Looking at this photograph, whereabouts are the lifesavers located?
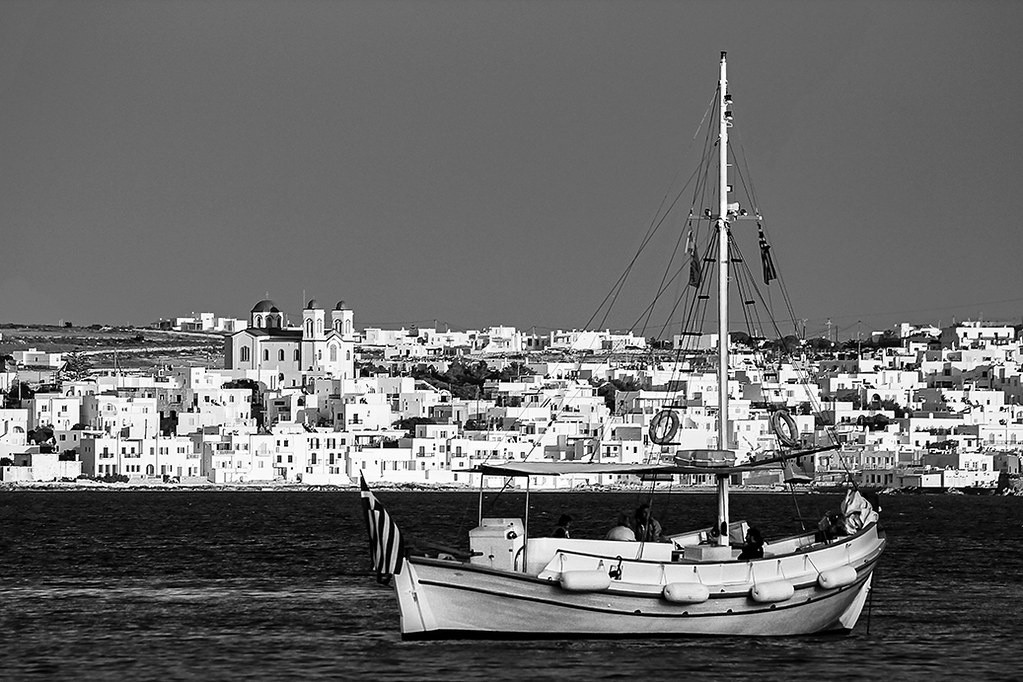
[649,410,679,443]
[773,410,799,445]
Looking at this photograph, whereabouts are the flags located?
[359,477,404,575]
[689,233,703,288]
[758,225,777,285]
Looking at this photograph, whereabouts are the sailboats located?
[358,51,889,641]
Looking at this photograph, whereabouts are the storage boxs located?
[674,450,735,468]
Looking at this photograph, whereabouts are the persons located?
[606,515,636,541]
[737,528,764,561]
[637,504,662,543]
[552,513,574,539]
